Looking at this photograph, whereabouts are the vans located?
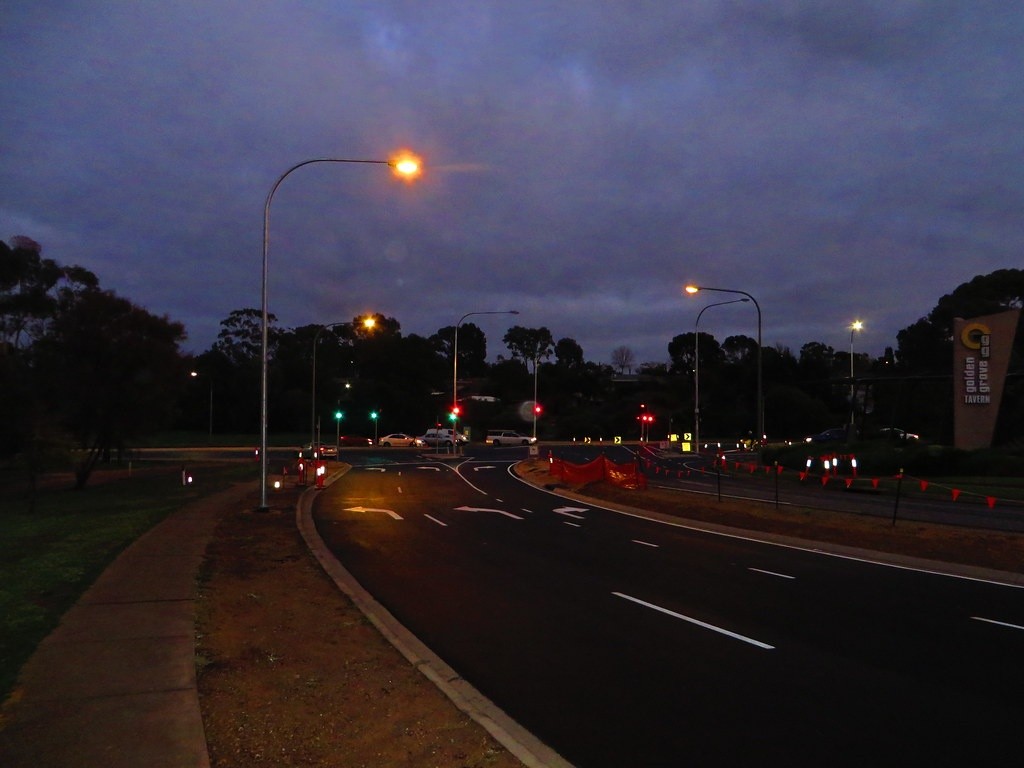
[427,428,469,445]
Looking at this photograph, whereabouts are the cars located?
[299,442,337,458]
[804,428,849,443]
[338,433,375,448]
[379,433,426,448]
[880,428,919,440]
[418,432,457,447]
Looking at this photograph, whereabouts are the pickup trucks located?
[486,431,536,447]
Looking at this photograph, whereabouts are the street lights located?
[310,319,377,461]
[695,298,751,452]
[643,416,653,443]
[335,412,342,443]
[685,285,762,448]
[453,311,519,455]
[191,372,212,436]
[851,322,861,424]
[257,156,417,512]
